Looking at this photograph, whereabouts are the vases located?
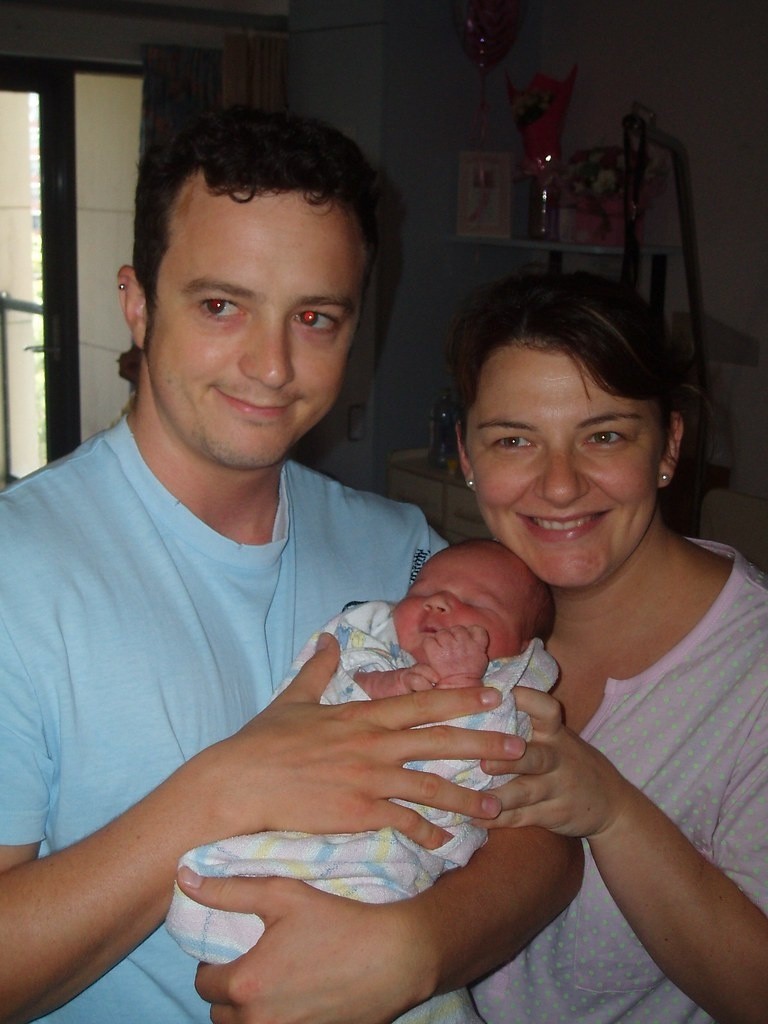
[526,178,558,235]
[575,211,645,246]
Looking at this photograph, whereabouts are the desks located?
[388,448,730,585]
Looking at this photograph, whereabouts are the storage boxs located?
[457,146,529,238]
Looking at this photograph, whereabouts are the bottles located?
[427,388,458,470]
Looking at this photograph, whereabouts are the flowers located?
[560,148,665,215]
[503,64,577,184]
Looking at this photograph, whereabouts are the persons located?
[168,538,564,967]
[0,104,585,1024]
[439,268,768,1024]
